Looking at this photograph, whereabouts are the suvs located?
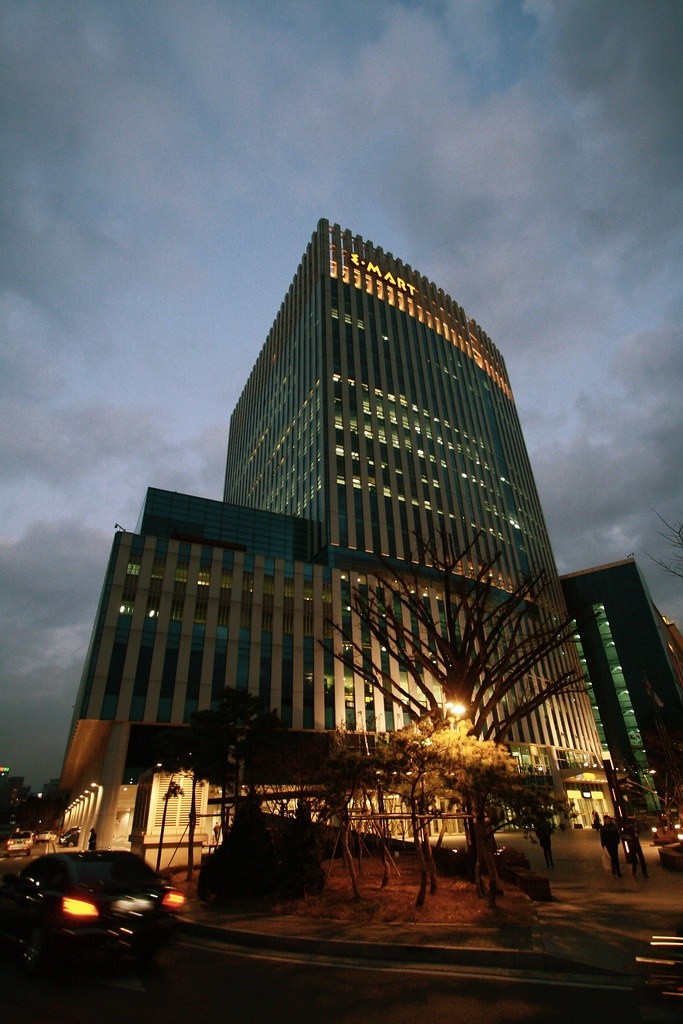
[6,830,33,858]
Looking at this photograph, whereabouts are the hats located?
[623,818,633,824]
[90,828,95,831]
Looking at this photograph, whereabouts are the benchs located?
[515,867,552,901]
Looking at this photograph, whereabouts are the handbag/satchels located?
[626,853,635,863]
[592,823,602,829]
[602,848,611,869]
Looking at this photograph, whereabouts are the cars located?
[59,826,82,848]
[35,831,57,844]
[653,821,683,845]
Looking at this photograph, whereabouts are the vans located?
[0,850,183,966]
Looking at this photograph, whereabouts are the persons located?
[213,822,221,842]
[533,810,652,879]
[88,828,96,850]
